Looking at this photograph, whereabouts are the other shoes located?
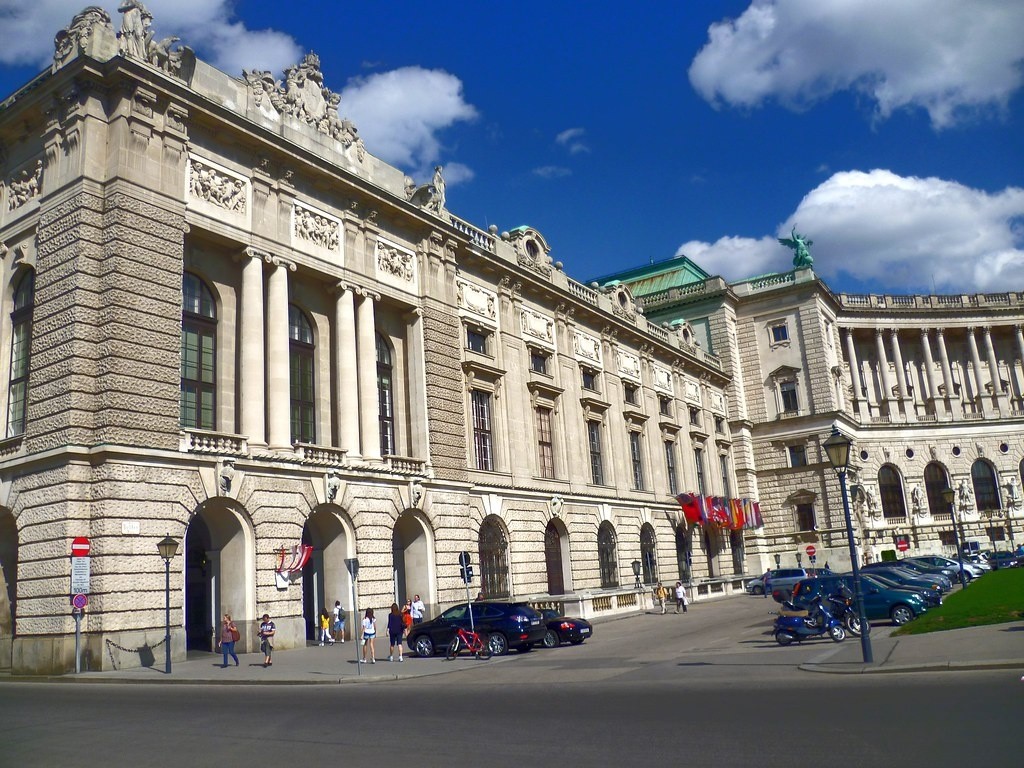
[674,611,679,614]
[684,612,686,613]
[221,665,227,668]
[329,638,335,642]
[360,659,366,663]
[683,611,686,613]
[236,660,239,666]
[399,656,403,662]
[268,662,272,666]
[372,659,375,663]
[390,656,393,661]
[329,642,333,646]
[264,663,267,668]
[341,639,344,643]
[319,644,324,646]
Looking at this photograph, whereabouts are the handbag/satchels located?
[684,598,689,605]
[231,622,240,642]
[401,622,407,629]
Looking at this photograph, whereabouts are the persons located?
[1007,477,1021,498]
[386,595,424,662]
[117,0,144,58]
[284,70,342,138]
[332,601,345,643]
[476,593,484,600]
[806,572,817,578]
[824,561,829,569]
[319,608,333,646]
[792,227,807,265]
[259,614,276,667]
[360,608,377,662]
[868,485,878,510]
[977,552,985,562]
[655,583,669,614]
[219,614,239,667]
[960,480,974,503]
[190,163,414,281]
[674,582,687,613]
[763,568,773,598]
[426,165,446,214]
[914,484,925,506]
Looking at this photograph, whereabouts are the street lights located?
[942,486,967,589]
[157,533,180,673]
[821,424,874,663]
[985,506,1000,569]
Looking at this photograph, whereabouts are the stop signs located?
[72,538,90,557]
[807,546,815,555]
[898,541,908,552]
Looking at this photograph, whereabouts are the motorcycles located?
[767,585,846,646]
[781,578,871,637]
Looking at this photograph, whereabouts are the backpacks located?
[336,607,345,621]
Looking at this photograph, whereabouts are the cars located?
[908,556,985,584]
[845,567,944,596]
[860,560,959,591]
[865,573,942,608]
[406,600,549,657]
[807,568,839,577]
[746,568,810,595]
[534,609,594,647]
[967,550,1019,573]
[792,576,929,626]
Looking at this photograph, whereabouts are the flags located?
[674,492,764,530]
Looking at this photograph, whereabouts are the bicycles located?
[446,623,494,661]
